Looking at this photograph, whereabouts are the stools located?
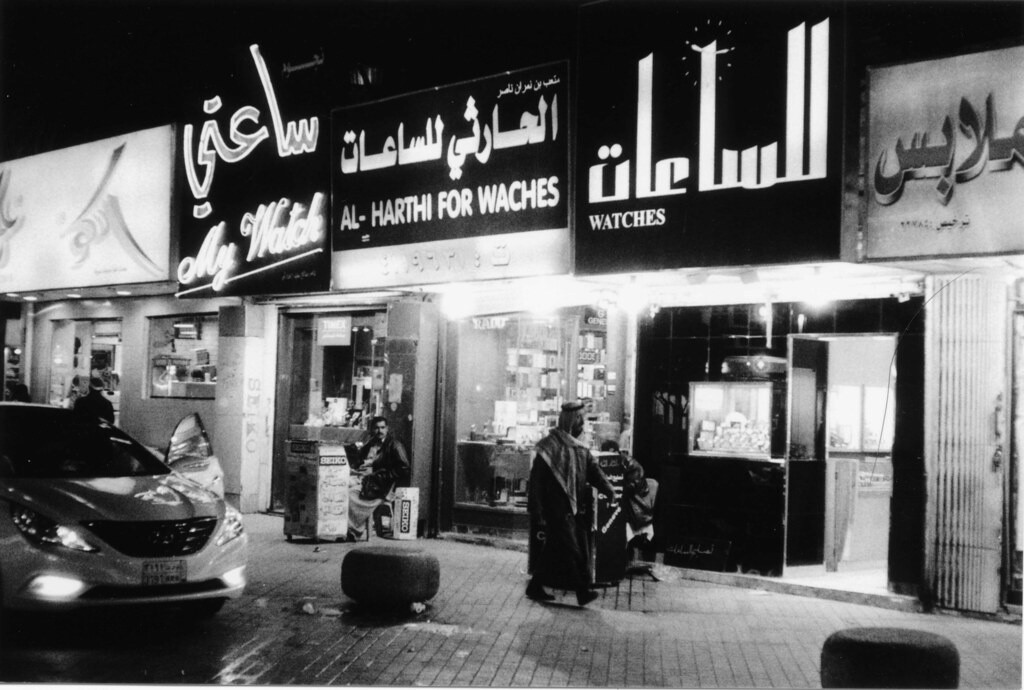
[820,626,961,690]
[340,545,439,614]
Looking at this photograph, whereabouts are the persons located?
[72,379,115,425]
[345,417,410,542]
[600,438,645,561]
[4,365,212,409]
[525,400,614,606]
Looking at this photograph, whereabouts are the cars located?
[0,398,251,625]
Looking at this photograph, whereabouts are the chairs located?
[350,481,399,542]
[623,478,659,582]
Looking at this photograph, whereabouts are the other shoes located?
[578,591,598,606]
[526,580,554,600]
